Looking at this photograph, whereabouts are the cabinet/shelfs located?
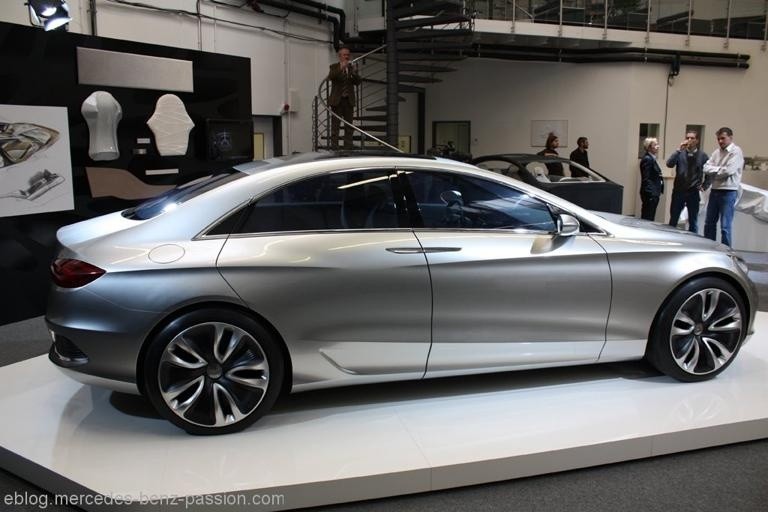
[533,0,713,36]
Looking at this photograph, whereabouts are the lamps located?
[27,0,71,33]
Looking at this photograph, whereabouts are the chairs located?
[340,179,397,229]
[533,167,552,183]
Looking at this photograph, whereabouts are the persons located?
[536,131,565,177]
[443,140,456,155]
[638,136,664,221]
[702,126,744,248]
[326,46,361,151]
[568,136,589,178]
[665,128,712,234]
